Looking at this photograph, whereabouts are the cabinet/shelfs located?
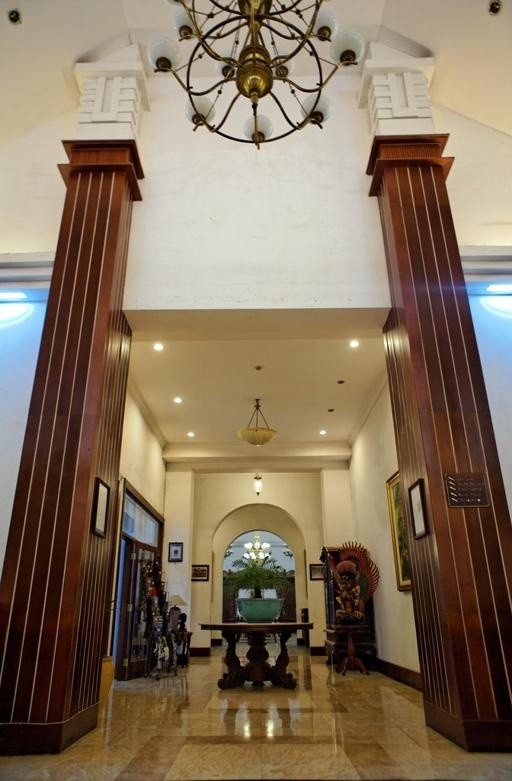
[319,545,377,664]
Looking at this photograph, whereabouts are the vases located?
[98,656,114,704]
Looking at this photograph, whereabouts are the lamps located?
[167,594,188,630]
[242,532,272,567]
[153,0,359,152]
[253,472,263,495]
[237,398,278,446]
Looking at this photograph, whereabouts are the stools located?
[331,623,372,674]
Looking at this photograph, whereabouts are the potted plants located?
[224,568,289,622]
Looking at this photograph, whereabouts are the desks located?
[173,631,193,668]
[198,622,314,688]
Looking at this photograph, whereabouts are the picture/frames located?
[408,477,430,539]
[90,476,111,538]
[168,541,183,561]
[192,564,208,580]
[310,563,326,579]
[386,469,416,591]
